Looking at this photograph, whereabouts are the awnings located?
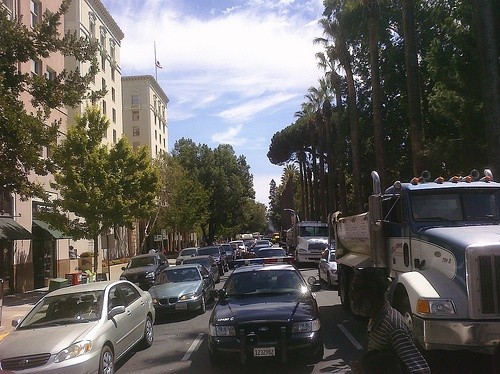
[0,216,33,240]
[33,220,73,239]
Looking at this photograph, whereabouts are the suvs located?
[120,253,170,292]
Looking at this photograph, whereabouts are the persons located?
[69,246,76,259]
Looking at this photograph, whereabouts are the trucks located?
[330,170,500,353]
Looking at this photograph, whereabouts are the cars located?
[206,254,324,366]
[175,207,344,285]
[316,248,339,287]
[0,280,156,374]
[147,263,215,315]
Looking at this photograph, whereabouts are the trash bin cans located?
[95,272,108,281]
